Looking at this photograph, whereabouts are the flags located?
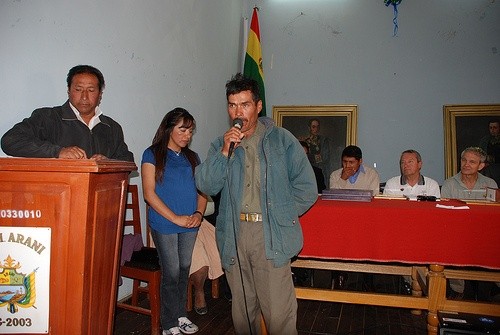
[244,7,267,117]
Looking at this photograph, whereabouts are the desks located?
[260,195,500,335]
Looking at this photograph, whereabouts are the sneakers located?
[162,327,182,335]
[178,317,198,334]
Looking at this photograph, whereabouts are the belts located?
[240,213,263,222]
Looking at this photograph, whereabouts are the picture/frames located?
[272,105,358,195]
[442,104,500,188]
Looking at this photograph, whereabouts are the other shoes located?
[194,306,208,314]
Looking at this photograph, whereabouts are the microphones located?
[228,118,243,159]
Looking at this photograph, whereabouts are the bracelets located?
[193,211,202,216]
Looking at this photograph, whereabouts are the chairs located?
[187,275,219,312]
[115,184,161,335]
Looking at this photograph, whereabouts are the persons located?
[383,150,441,201]
[142,108,207,335]
[0,65,134,162]
[331,145,380,197]
[195,73,318,335]
[299,141,326,193]
[189,218,224,315]
[441,148,498,298]
